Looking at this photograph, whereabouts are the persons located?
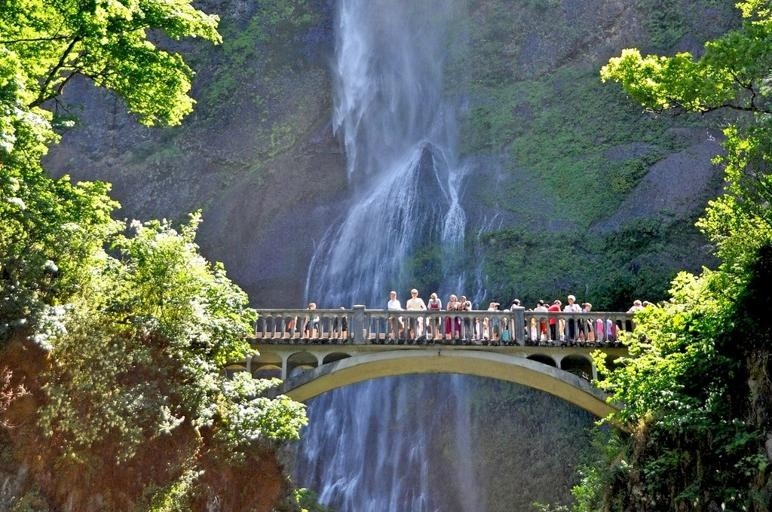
[625,299,643,331]
[445,294,620,342]
[305,302,319,339]
[387,290,404,339]
[642,300,655,308]
[333,307,348,338]
[406,289,427,339]
[427,292,442,339]
[288,317,297,337]
